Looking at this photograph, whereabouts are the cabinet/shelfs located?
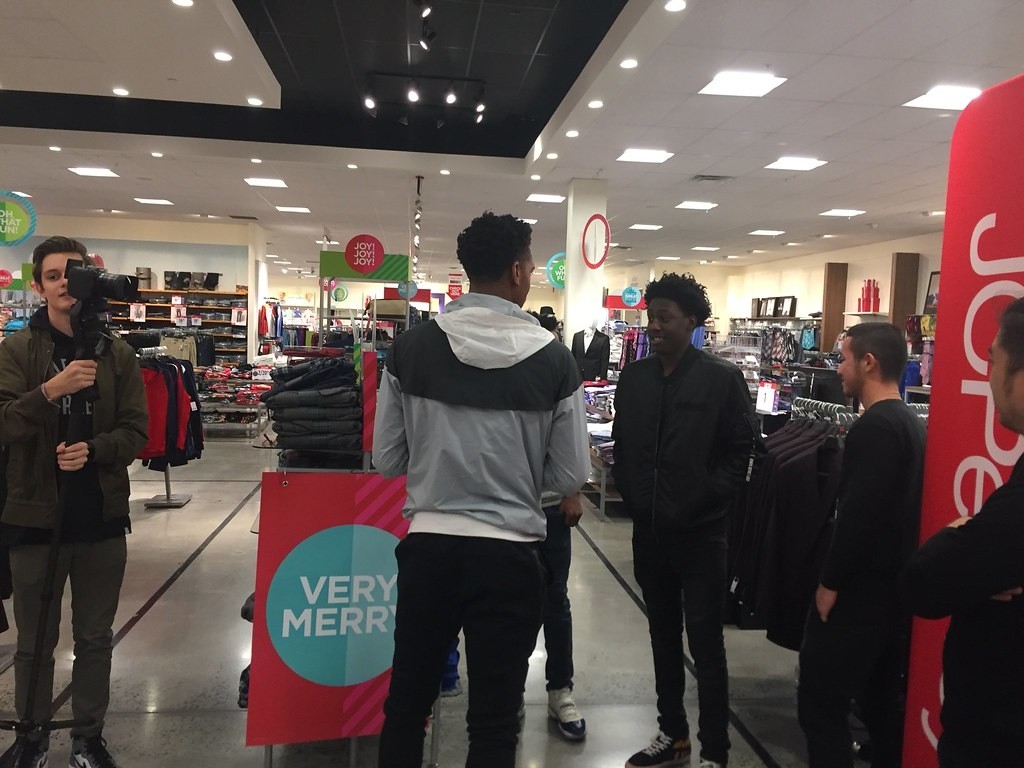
[581,317,933,523]
[0,279,413,440]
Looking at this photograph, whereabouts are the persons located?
[570,309,610,384]
[897,295,1024,768]
[477,311,587,740]
[794,321,929,768]
[370,211,592,766]
[611,272,765,768]
[0,235,151,768]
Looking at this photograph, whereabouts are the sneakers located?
[627,728,692,767]
[548,685,585,738]
[696,758,727,768]
[7,736,50,767]
[68,737,116,768]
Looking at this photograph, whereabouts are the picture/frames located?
[923,270,940,315]
[752,296,794,317]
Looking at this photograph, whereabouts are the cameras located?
[65,259,138,359]
